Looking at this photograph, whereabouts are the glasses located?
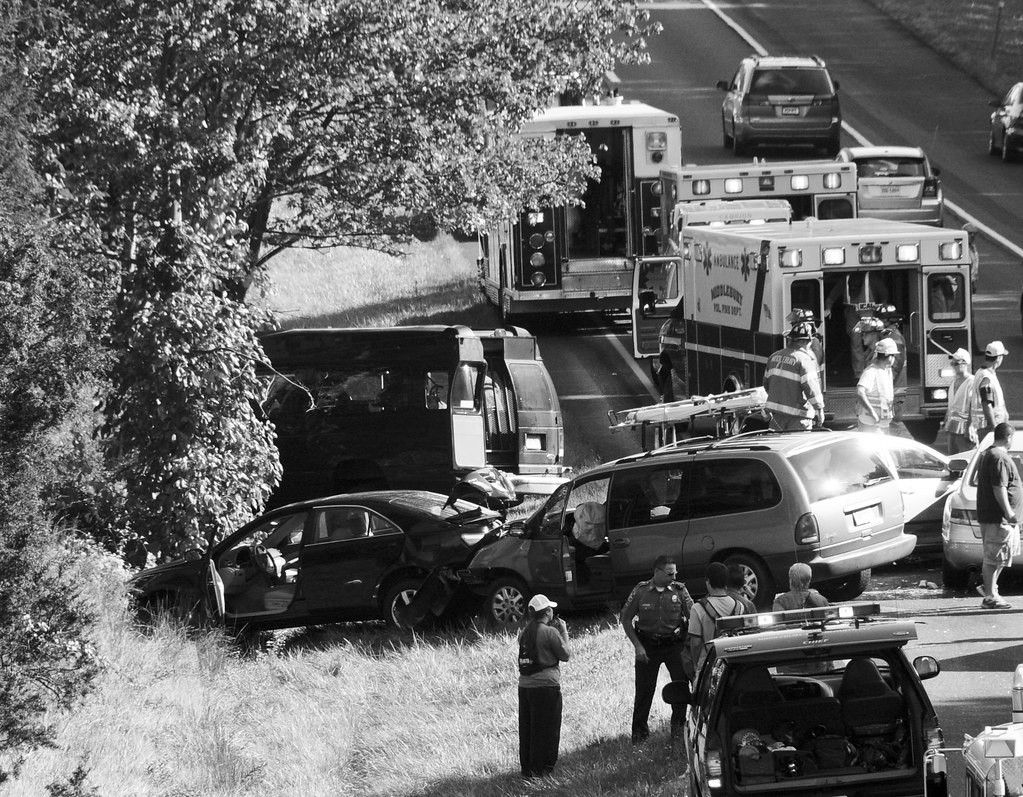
[656,566,678,576]
[950,361,966,366]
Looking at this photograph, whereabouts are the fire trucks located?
[477,78,682,331]
[632,201,977,444]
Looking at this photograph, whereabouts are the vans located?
[248,323,565,519]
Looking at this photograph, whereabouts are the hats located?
[984,341,1009,357]
[948,347,971,364]
[529,593,557,613]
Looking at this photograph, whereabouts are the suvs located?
[463,428,918,634]
[715,53,841,156]
[835,146,945,228]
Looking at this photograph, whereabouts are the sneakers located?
[982,596,1012,609]
[976,584,1000,598]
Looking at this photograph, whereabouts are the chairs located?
[839,659,901,724]
[341,517,367,537]
[739,670,785,706]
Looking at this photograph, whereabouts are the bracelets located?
[561,632,568,636]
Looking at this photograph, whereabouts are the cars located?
[941,426,1023,584]
[124,489,504,647]
[866,433,958,563]
[662,619,944,797]
[988,82,1023,160]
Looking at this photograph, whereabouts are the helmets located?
[874,338,901,356]
[785,323,817,339]
[874,302,896,317]
[848,316,884,336]
[784,308,814,323]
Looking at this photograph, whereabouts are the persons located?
[773,564,834,673]
[857,338,901,437]
[688,563,757,672]
[942,348,975,456]
[518,594,572,777]
[976,422,1023,609]
[962,223,985,356]
[969,341,1009,443]
[621,555,694,745]
[825,271,907,422]
[764,308,825,432]
